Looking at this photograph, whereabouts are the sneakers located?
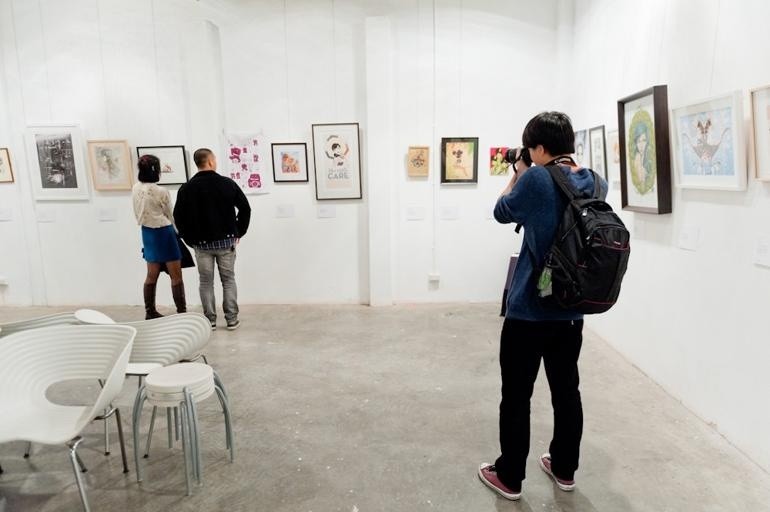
[227,319,240,329]
[540,453,576,492]
[211,321,217,330]
[478,462,522,501]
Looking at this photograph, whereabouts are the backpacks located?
[526,165,630,315]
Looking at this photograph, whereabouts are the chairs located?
[0,311,129,458]
[0,325,136,512]
[74,309,225,458]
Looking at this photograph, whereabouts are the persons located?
[477,111,609,500]
[633,122,650,182]
[133,154,186,320]
[173,148,251,330]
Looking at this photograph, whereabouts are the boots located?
[171,281,186,313]
[144,283,165,319]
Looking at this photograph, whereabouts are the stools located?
[131,363,235,496]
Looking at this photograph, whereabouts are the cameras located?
[504,147,533,167]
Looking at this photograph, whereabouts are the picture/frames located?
[220,125,269,197]
[270,142,310,184]
[441,136,478,187]
[570,85,770,214]
[24,127,87,202]
[406,145,431,180]
[85,139,136,193]
[0,147,16,187]
[311,124,363,201]
[136,144,190,186]
